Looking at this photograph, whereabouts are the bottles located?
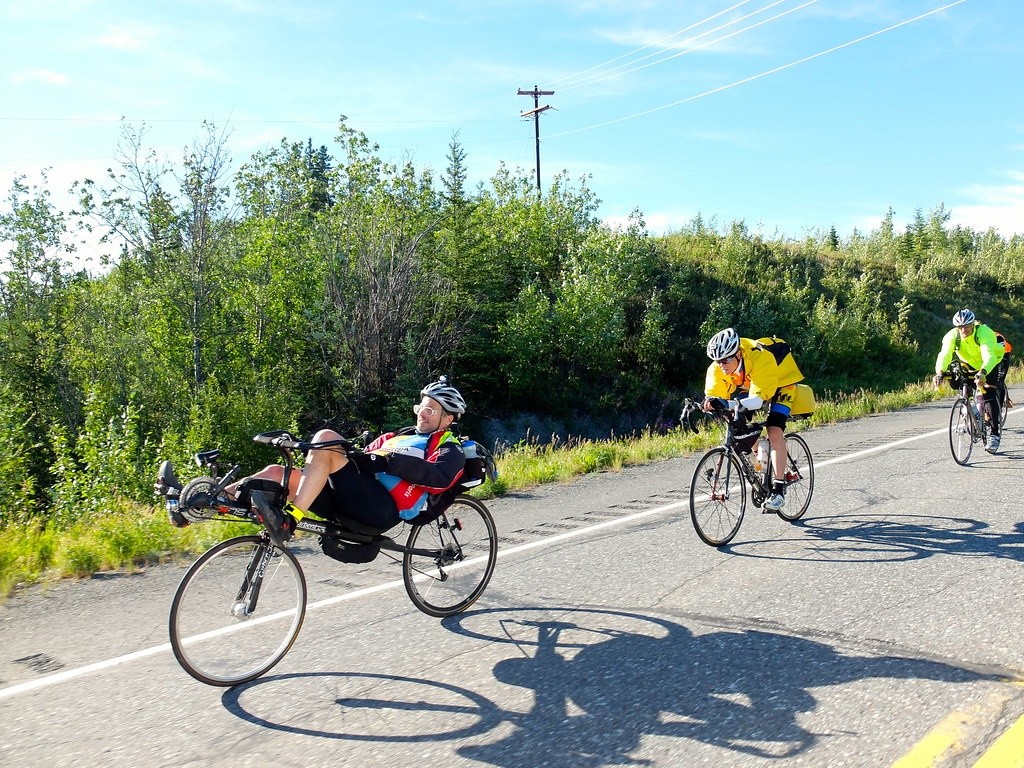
[976,395,983,417]
[747,448,760,471]
[970,399,980,418]
[757,437,769,473]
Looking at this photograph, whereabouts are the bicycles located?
[169,430,498,687]
[679,384,816,547]
[935,360,1013,465]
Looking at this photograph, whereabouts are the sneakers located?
[761,493,786,511]
[252,488,297,549]
[158,461,193,529]
[986,434,1001,450]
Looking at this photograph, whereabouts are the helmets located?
[420,375,466,414]
[706,328,739,361]
[952,309,975,327]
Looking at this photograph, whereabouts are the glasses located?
[413,404,451,418]
[715,355,736,365]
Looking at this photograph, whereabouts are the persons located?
[153,381,466,547]
[699,328,805,511]
[933,309,1012,452]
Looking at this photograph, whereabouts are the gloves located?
[346,449,387,476]
